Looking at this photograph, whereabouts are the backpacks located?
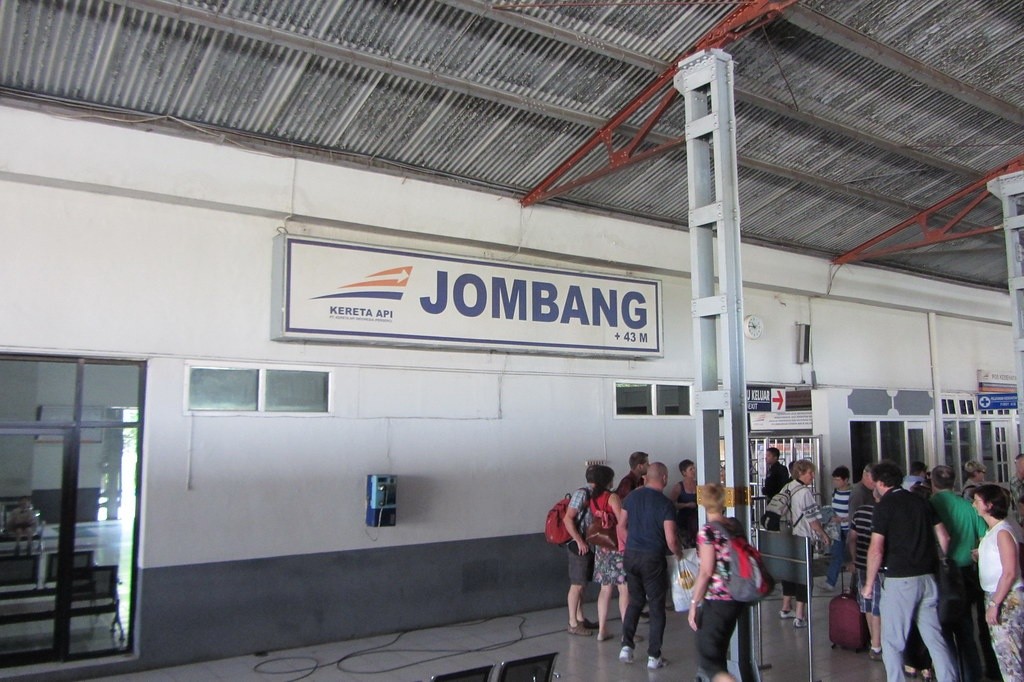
[710,516,774,601]
[545,487,590,544]
[761,482,807,534]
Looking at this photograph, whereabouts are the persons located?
[589,466,643,643]
[615,451,650,624]
[779,459,831,628]
[687,484,737,682]
[671,459,698,531]
[562,464,599,637]
[618,462,684,670]
[787,453,1024,682]
[861,461,957,682]
[761,447,789,506]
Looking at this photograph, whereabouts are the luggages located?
[830,565,869,653]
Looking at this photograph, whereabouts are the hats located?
[965,460,987,473]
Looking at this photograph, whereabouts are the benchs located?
[419,650,560,682]
[0,500,126,644]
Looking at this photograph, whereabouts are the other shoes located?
[817,582,835,592]
[665,599,674,611]
[637,611,650,625]
[596,632,614,641]
[903,664,917,679]
[981,665,1003,681]
[621,633,643,642]
[920,668,933,682]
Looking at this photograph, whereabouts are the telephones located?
[365,474,398,529]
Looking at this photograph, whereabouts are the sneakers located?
[793,615,807,628]
[779,608,796,618]
[647,654,668,669]
[619,645,634,664]
[869,645,883,661]
[567,622,593,636]
[577,617,598,628]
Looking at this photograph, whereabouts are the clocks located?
[745,315,763,339]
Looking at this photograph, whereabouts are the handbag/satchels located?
[665,548,705,613]
[678,508,698,532]
[586,491,618,551]
[937,557,965,612]
[823,507,842,542]
